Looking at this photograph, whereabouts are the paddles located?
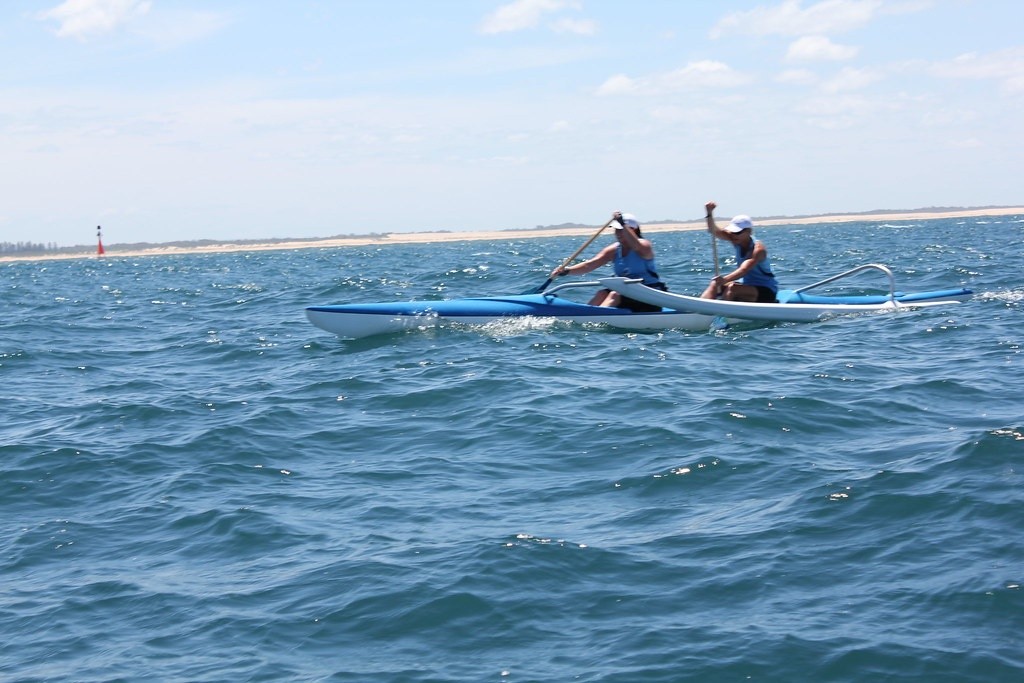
[518,219,614,296]
[711,207,719,277]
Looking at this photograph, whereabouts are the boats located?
[305,278,741,338]
[597,264,974,320]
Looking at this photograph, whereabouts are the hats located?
[609,213,638,229]
[724,214,753,232]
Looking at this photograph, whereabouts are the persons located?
[700,203,778,303]
[550,211,667,312]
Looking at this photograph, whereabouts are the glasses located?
[732,228,748,235]
